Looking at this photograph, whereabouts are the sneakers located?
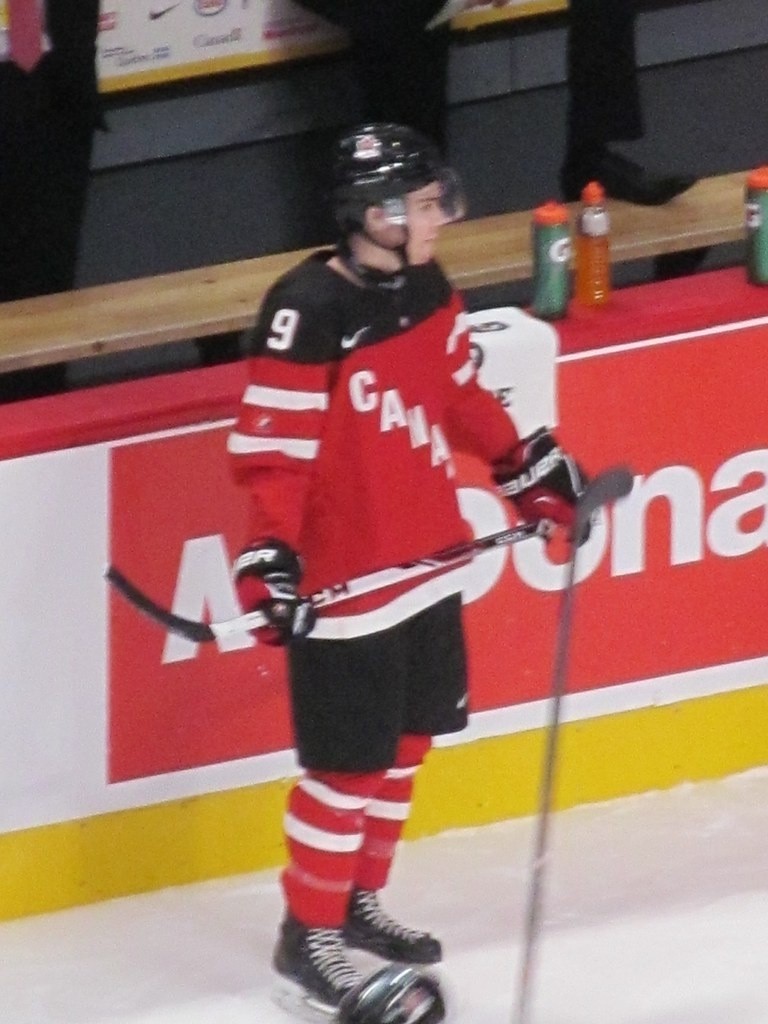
[340,884,443,965]
[267,908,364,1013]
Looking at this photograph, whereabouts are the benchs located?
[0,166,754,369]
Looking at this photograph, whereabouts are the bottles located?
[574,178,617,309]
[527,201,574,319]
[743,163,768,287]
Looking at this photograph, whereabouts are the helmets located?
[333,959,451,1024]
[332,121,443,223]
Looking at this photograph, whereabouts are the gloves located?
[230,535,318,648]
[491,424,594,565]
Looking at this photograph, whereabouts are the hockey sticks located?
[512,457,634,1024]
[101,463,605,643]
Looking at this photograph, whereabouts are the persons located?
[227,123,590,1024]
[303,0,697,207]
[0,0,106,403]
